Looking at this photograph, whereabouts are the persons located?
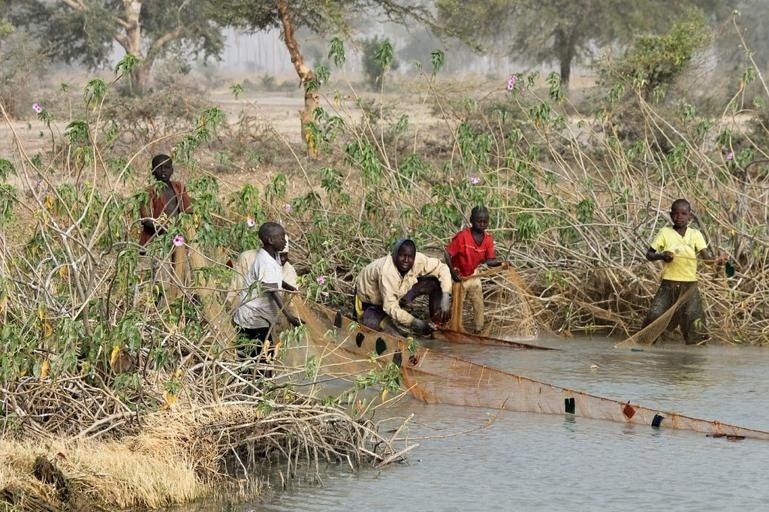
[641,199,730,346]
[138,154,194,257]
[353,234,453,337]
[442,204,509,332]
[232,221,308,375]
[224,225,299,334]
